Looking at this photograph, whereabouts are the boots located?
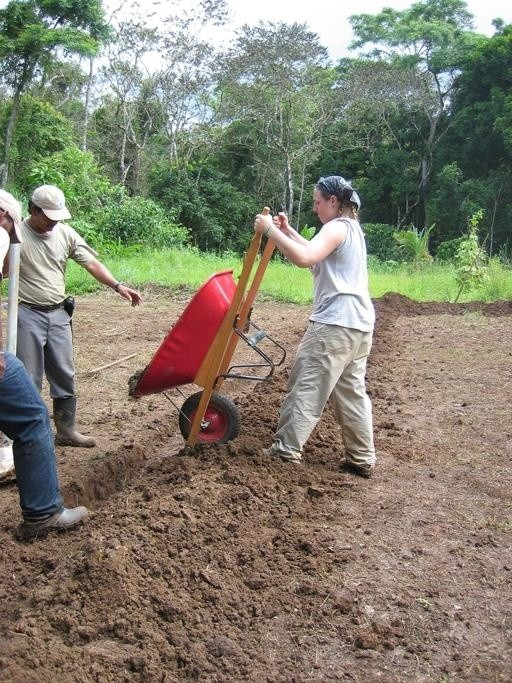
[52,393,98,448]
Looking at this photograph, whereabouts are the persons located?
[5,183,144,450]
[252,173,379,476]
[0,188,96,540]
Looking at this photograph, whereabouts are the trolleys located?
[134,207,286,448]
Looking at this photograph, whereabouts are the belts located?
[18,296,68,313]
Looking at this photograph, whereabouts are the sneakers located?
[25,504,90,537]
[338,456,372,479]
[263,444,301,464]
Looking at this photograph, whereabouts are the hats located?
[32,183,73,221]
[0,187,25,244]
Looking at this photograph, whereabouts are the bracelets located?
[114,281,124,293]
[264,225,272,239]
